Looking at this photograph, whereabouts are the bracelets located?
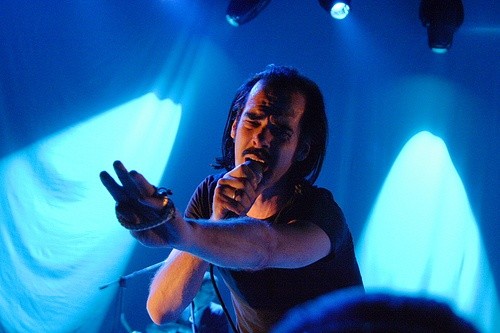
[116,203,175,230]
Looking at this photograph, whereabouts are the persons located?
[100,65,365,333]
[147,272,228,333]
[273,291,481,333]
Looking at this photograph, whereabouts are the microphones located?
[221,161,264,220]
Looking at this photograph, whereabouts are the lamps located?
[418,0,465,54]
[225,0,271,28]
[318,0,351,20]
